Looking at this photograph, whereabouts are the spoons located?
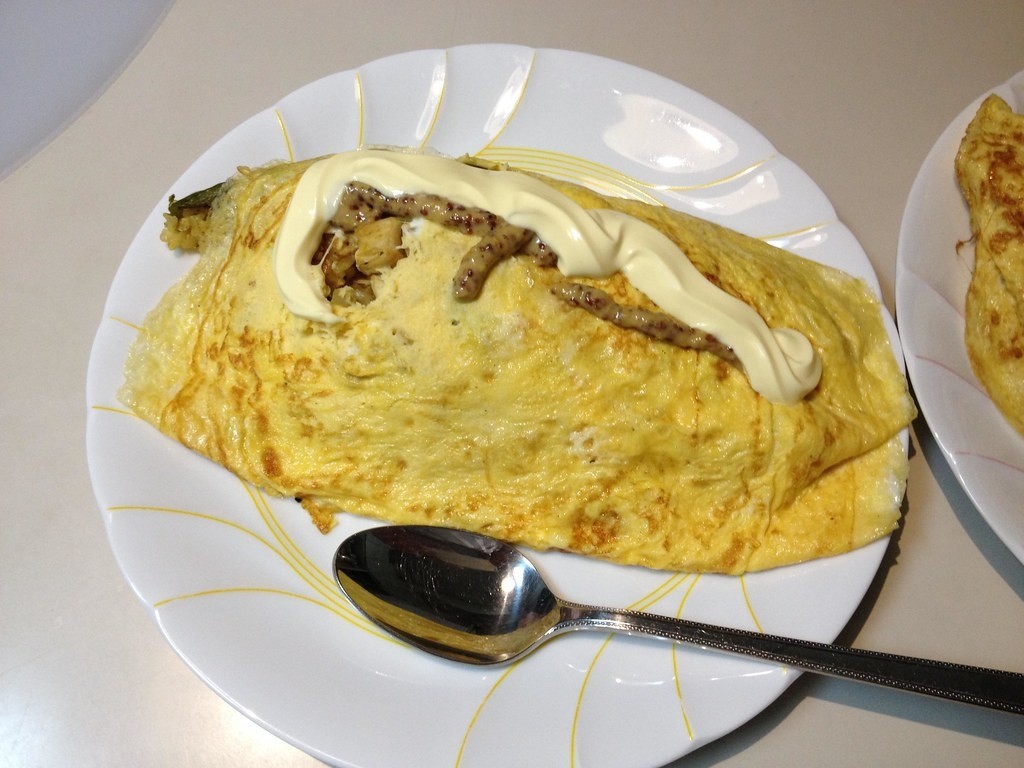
[335,527,1022,720]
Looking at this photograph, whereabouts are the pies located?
[117,73,1024,612]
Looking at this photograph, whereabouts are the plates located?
[87,43,917,768]
[897,65,1022,566]
